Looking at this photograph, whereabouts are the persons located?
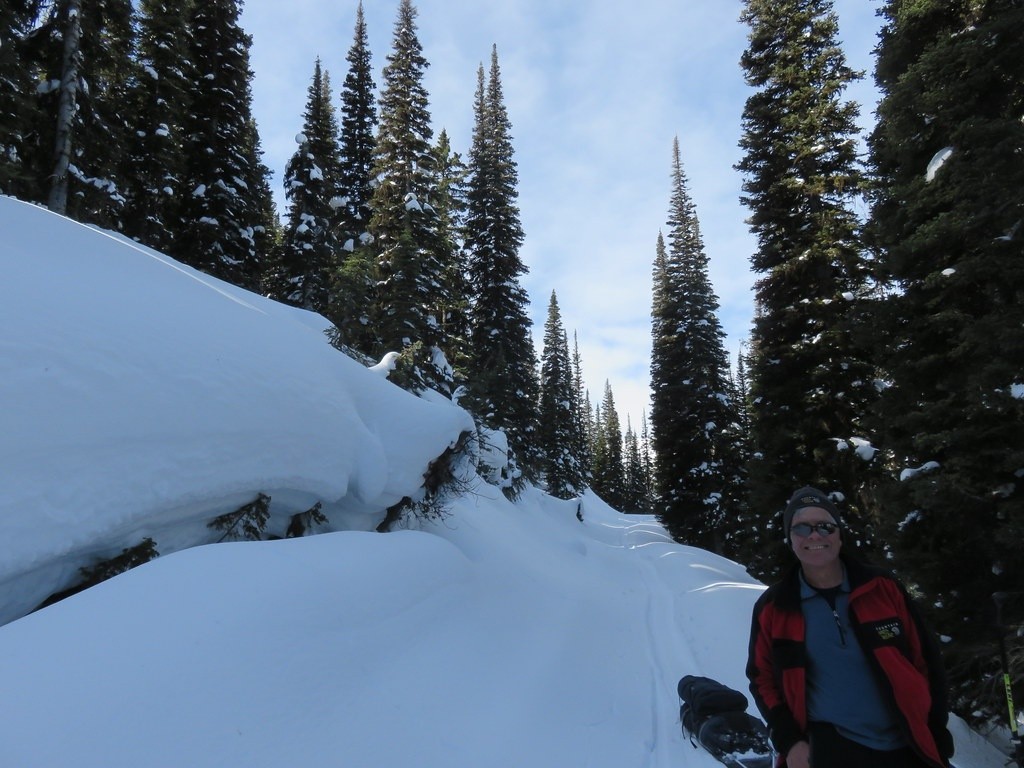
[745,486,956,768]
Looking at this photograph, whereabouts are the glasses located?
[789,522,840,538]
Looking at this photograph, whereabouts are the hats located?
[783,486,845,544]
